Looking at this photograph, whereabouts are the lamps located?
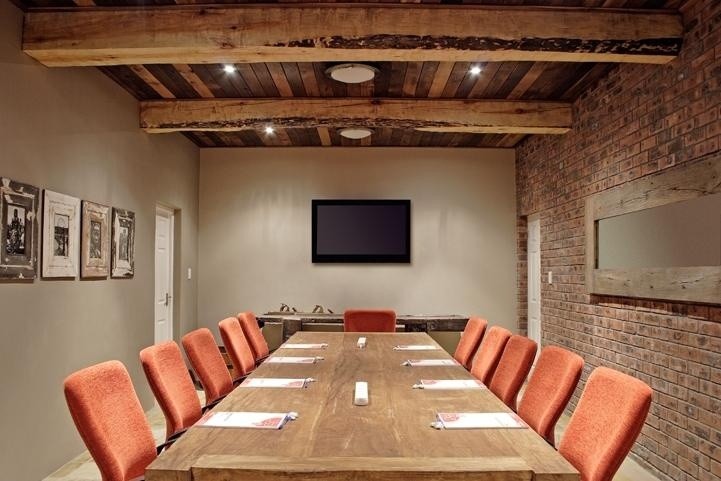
[327,63,382,84]
[334,127,373,139]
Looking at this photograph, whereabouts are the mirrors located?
[581,150,721,309]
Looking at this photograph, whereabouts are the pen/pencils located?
[279,417,290,429]
[304,381,308,388]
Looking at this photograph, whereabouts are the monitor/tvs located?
[311,199,411,265]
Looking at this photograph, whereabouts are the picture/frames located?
[42,187,80,281]
[112,205,136,280]
[0,176,38,284]
[82,197,110,280]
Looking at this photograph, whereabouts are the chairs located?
[553,365,654,481]
[235,309,279,367]
[452,316,539,414]
[517,342,587,451]
[180,327,246,414]
[216,317,270,388]
[138,337,222,452]
[62,360,178,481]
[341,308,397,332]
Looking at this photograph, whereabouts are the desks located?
[256,313,471,357]
[145,330,580,480]
